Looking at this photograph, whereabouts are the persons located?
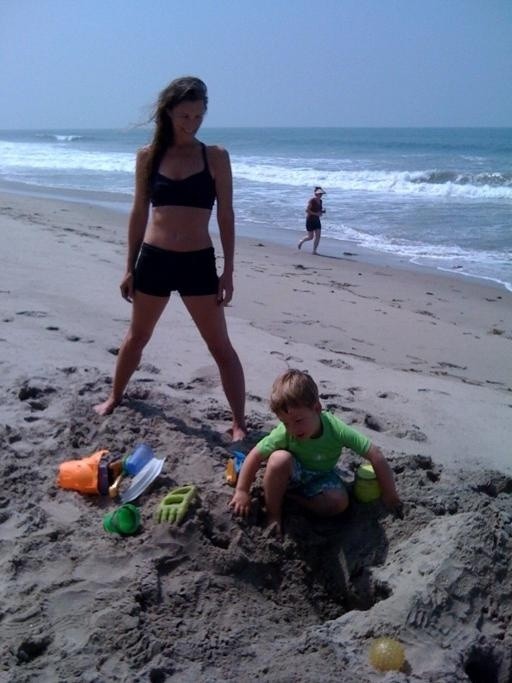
[228,367,403,541]
[91,76,249,444]
[298,187,327,256]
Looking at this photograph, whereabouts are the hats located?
[315,189,326,194]
